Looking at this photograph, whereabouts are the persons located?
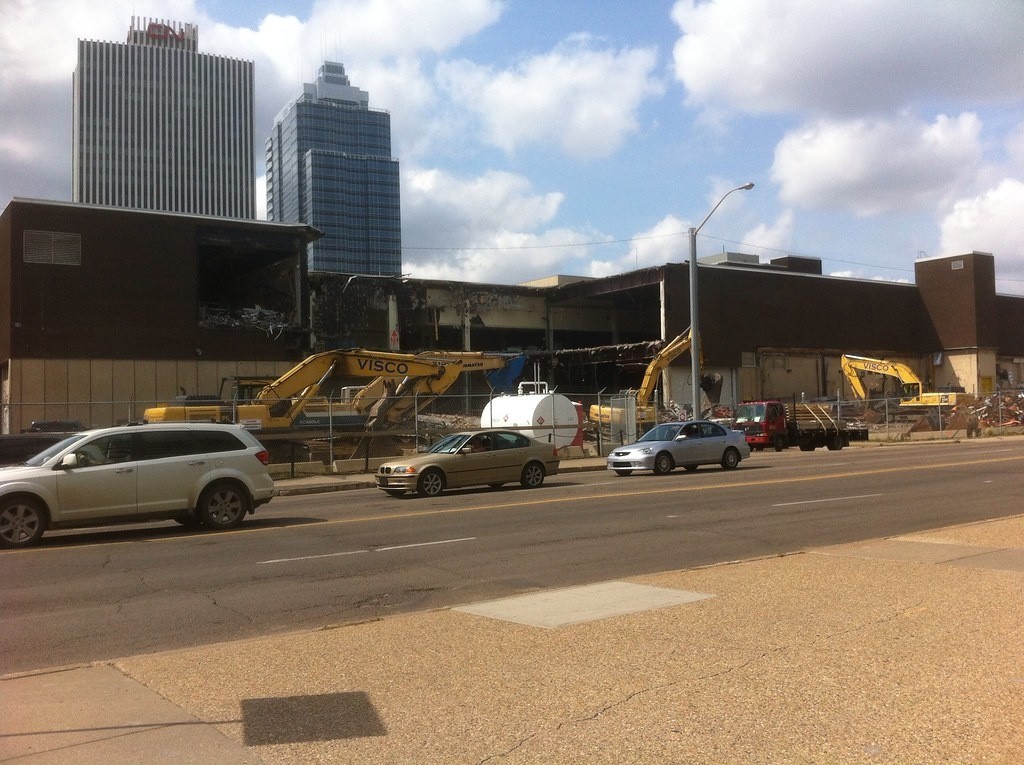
[473,438,485,452]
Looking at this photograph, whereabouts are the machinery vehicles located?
[145,346,522,460]
[841,354,976,431]
[588,324,724,430]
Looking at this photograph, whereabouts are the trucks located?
[735,400,869,451]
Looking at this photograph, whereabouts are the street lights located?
[688,183,755,415]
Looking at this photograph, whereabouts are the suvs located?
[1,423,273,550]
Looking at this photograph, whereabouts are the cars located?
[608,421,751,474]
[374,432,561,497]
